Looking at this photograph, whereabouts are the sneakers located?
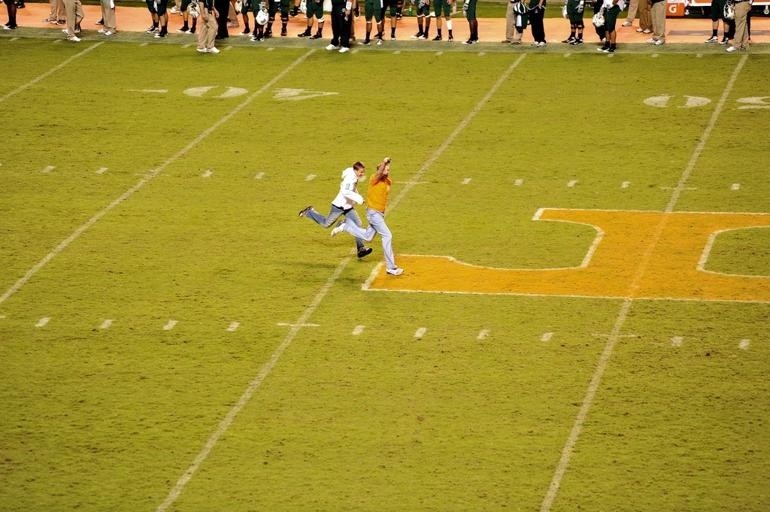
[95,19,116,35]
[309,34,322,39]
[325,31,396,54]
[386,266,404,276]
[501,39,547,49]
[147,25,169,39]
[357,246,372,258]
[330,218,348,238]
[298,32,311,37]
[299,206,315,217]
[1,23,17,30]
[410,32,479,45]
[40,18,67,26]
[622,20,666,45]
[61,24,82,42]
[176,26,196,34]
[214,22,288,44]
[704,35,752,53]
[561,36,619,54]
[196,46,212,53]
[206,47,221,54]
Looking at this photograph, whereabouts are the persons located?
[412,0,479,45]
[3,0,17,30]
[502,0,546,47]
[298,161,373,258]
[705,0,752,52]
[561,0,666,53]
[330,155,405,277]
[96,0,116,36]
[146,0,405,54]
[47,0,84,41]
